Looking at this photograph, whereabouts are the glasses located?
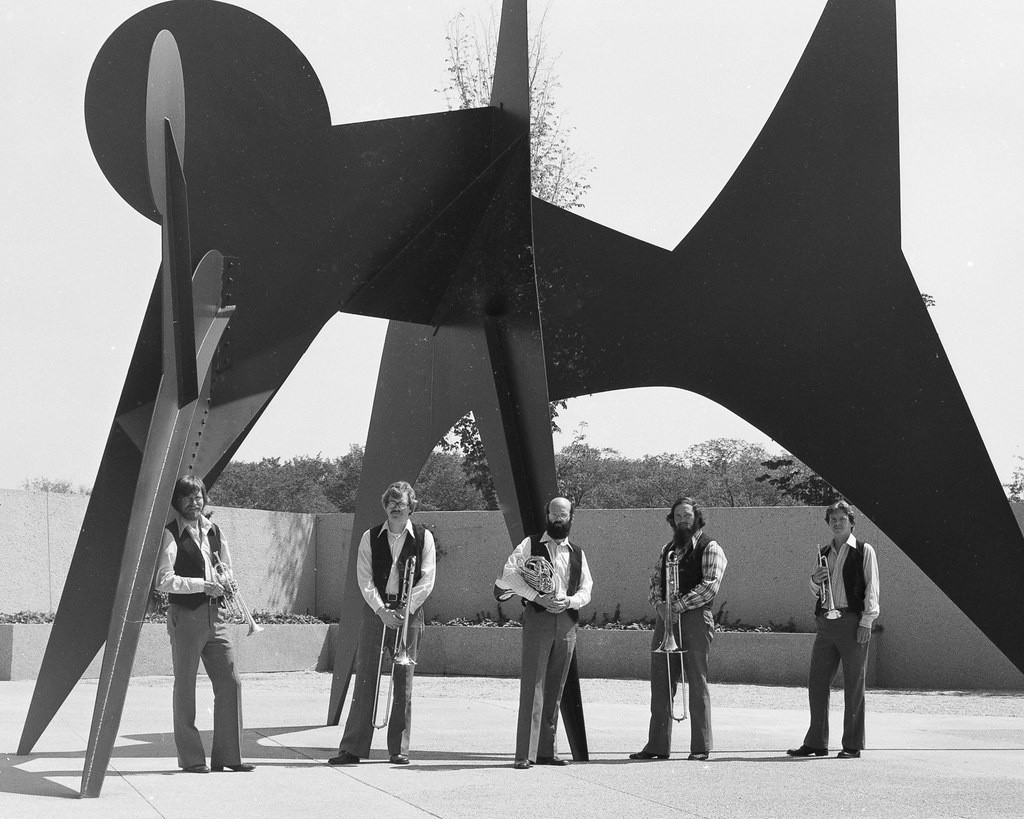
[548,511,571,520]
[386,500,411,510]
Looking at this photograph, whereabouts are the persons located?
[155,475,256,773]
[630,499,727,760]
[327,480,436,768]
[788,499,880,758]
[502,497,593,771]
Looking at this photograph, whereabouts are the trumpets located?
[209,551,265,637]
[816,544,842,620]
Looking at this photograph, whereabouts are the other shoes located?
[629,751,670,761]
[688,751,709,762]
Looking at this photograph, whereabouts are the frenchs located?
[493,556,556,602]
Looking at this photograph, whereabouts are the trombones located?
[650,550,689,722]
[371,556,418,729]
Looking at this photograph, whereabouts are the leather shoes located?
[514,757,531,769]
[390,754,410,765]
[837,748,861,760]
[538,757,570,765]
[328,751,360,764]
[184,766,210,773]
[211,763,256,772]
[787,744,829,757]
